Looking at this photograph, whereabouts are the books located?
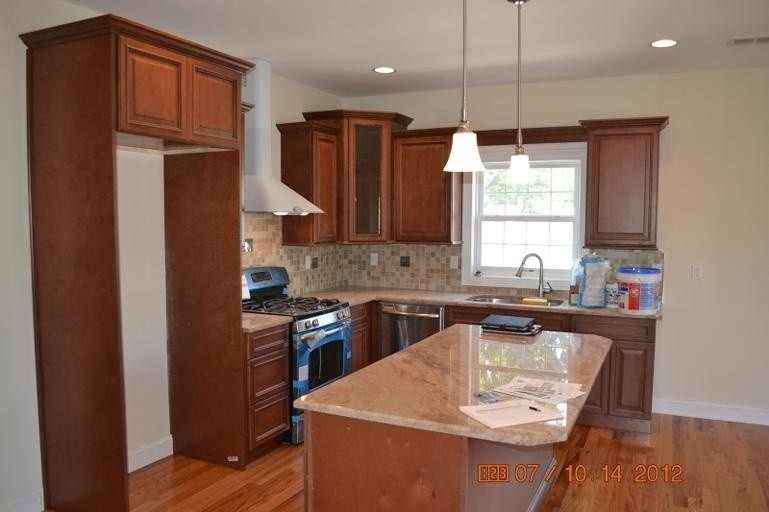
[458,374,587,431]
[478,312,543,337]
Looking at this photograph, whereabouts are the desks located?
[294,321,613,512]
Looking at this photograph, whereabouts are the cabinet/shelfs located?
[277,122,337,245]
[392,129,458,242]
[347,300,374,369]
[302,108,415,245]
[168,313,289,471]
[568,314,656,429]
[579,116,668,246]
[19,14,256,149]
[374,286,444,360]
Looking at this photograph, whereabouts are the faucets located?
[516,253,553,299]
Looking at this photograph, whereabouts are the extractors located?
[239,60,325,216]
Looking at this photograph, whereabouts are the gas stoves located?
[242,266,351,332]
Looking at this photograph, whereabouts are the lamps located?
[505,2,534,180]
[443,0,487,173]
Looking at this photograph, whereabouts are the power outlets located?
[450,256,459,269]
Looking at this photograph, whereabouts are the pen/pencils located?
[529,406,541,412]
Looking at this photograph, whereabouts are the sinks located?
[465,295,564,307]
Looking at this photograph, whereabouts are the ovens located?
[280,319,352,445]
